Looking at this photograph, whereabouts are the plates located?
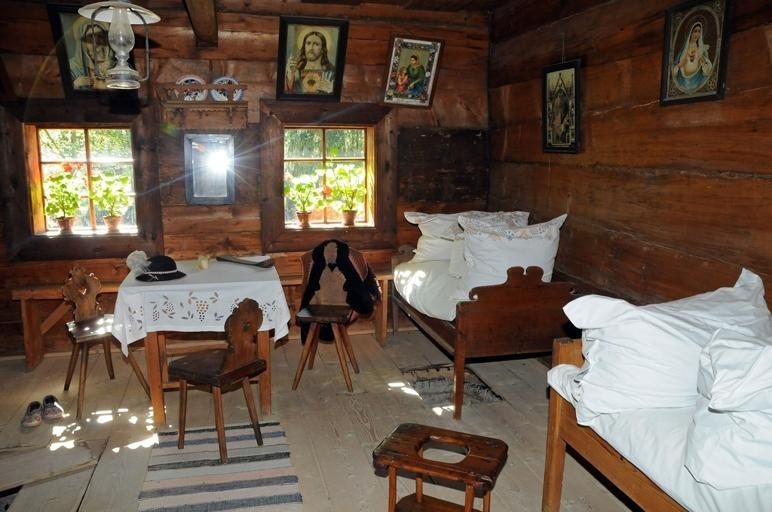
[211,76,242,103]
[174,74,208,102]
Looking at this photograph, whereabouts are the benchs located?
[270,246,395,348]
[8,257,164,375]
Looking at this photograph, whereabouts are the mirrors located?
[185,133,237,205]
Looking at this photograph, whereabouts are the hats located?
[136,255,186,282]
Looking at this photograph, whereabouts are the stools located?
[373,422,508,512]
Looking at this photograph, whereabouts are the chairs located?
[290,239,383,392]
[167,297,267,465]
[62,263,154,422]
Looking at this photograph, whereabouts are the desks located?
[112,255,289,425]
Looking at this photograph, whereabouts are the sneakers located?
[21,400,44,428]
[42,395,64,420]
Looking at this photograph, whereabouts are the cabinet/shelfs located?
[158,83,249,135]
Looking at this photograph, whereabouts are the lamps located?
[77,0,161,89]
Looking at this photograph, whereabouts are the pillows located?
[405,207,570,282]
[563,266,771,421]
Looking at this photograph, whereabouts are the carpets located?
[136,419,305,512]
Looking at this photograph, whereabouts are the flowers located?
[41,161,81,216]
[88,169,129,216]
[323,169,368,211]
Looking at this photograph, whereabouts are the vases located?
[101,216,121,231]
[53,216,76,232]
[341,211,358,226]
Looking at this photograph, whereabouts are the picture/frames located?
[659,0,734,106]
[277,14,350,102]
[379,31,446,109]
[541,58,582,153]
[45,2,138,100]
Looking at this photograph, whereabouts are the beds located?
[542,335,772,511]
[390,247,593,419]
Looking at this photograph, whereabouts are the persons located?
[402,54,424,97]
[671,21,713,93]
[550,90,567,129]
[395,65,408,94]
[66,19,116,89]
[286,28,336,93]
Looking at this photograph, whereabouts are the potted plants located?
[285,181,322,228]
